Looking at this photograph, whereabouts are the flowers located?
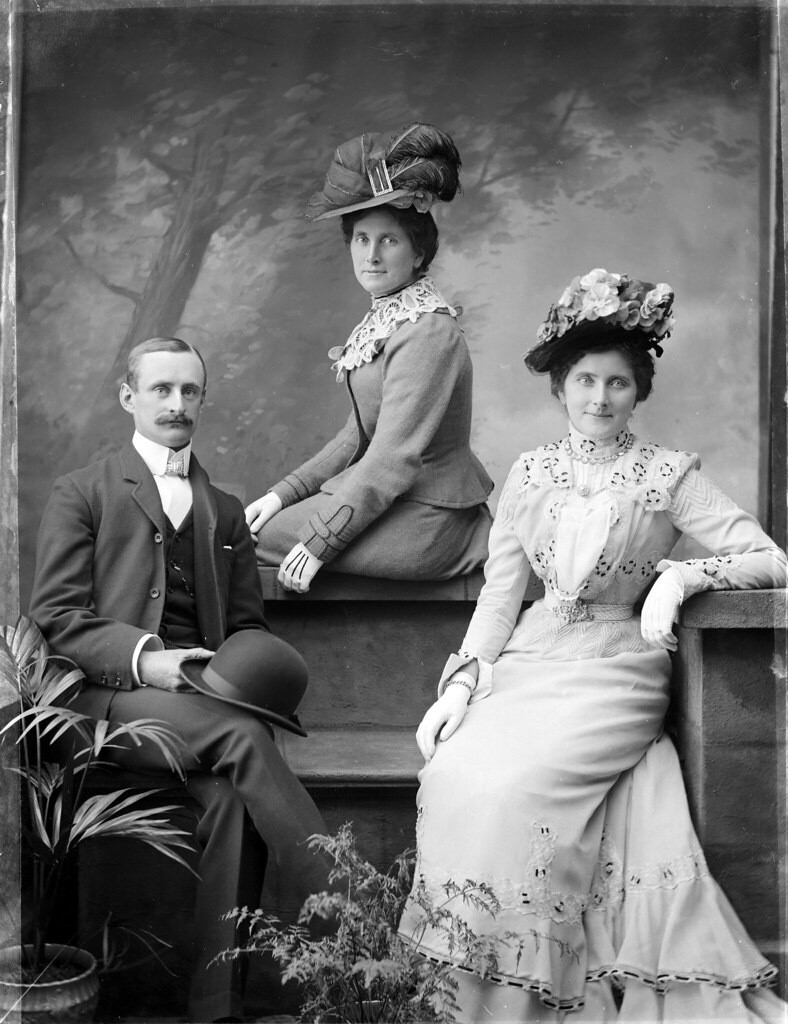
[537,268,678,357]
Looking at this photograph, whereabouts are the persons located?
[394,267,788,1024]
[237,124,495,596]
[28,333,364,1024]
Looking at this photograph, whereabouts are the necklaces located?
[565,435,633,465]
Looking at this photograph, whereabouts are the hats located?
[179,630,309,738]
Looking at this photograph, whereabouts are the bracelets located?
[442,678,473,694]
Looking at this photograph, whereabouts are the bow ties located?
[164,460,184,476]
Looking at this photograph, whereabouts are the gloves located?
[415,671,477,762]
[278,541,324,593]
[639,566,683,653]
[244,491,284,542]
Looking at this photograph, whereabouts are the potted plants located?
[207,820,579,1024]
[0,614,202,1024]
